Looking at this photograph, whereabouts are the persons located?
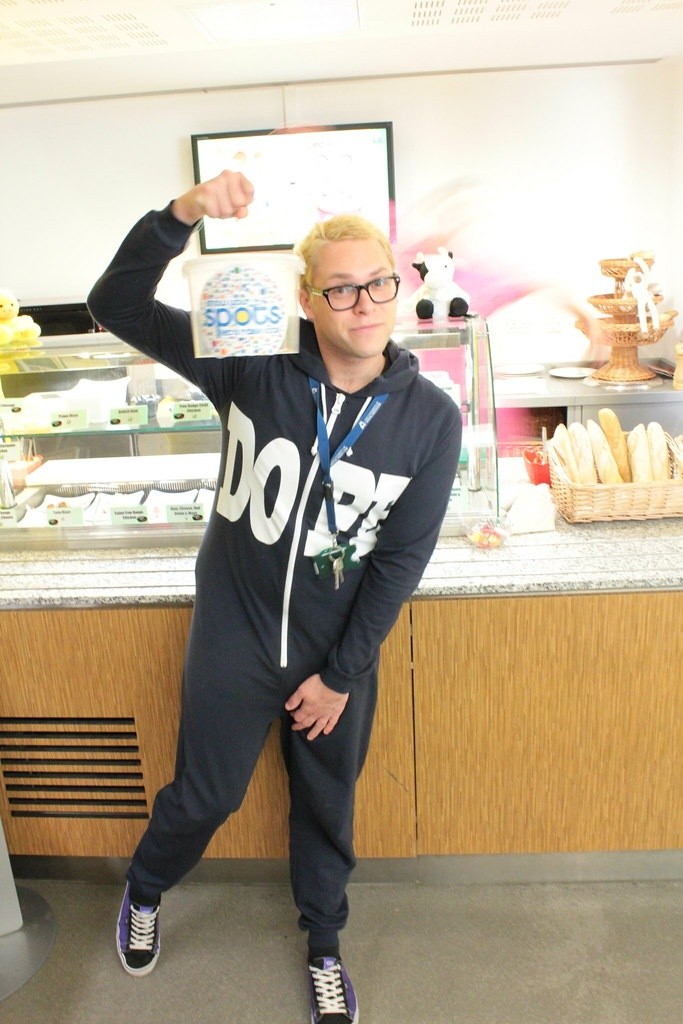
[85,171,461,1024]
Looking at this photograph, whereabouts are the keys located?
[333,557,344,591]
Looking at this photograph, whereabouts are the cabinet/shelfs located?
[0,310,498,553]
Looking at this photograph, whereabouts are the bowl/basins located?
[522,446,550,486]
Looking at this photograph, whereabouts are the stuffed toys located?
[412,245,470,319]
[0,286,41,346]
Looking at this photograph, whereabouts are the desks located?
[494,358,683,438]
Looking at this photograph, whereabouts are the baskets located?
[546,428,683,524]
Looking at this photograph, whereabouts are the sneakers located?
[306,948,360,1024]
[115,879,161,976]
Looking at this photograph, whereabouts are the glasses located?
[304,272,401,312]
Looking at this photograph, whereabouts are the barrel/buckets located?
[182,214,308,360]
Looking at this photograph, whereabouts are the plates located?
[549,367,597,379]
[493,363,546,377]
